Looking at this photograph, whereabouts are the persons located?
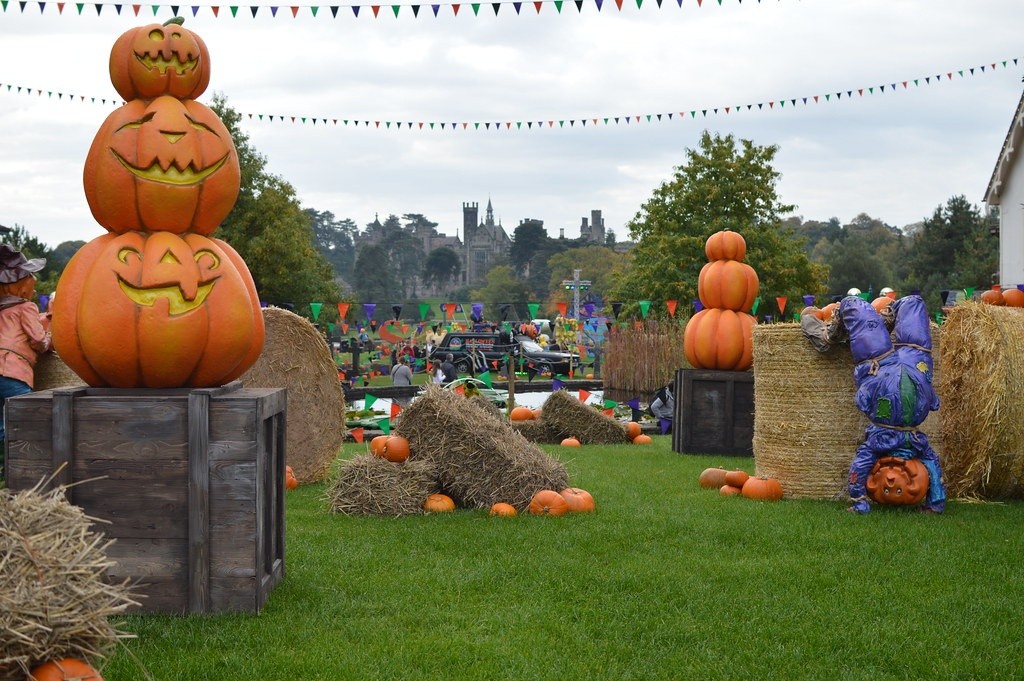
[536,334,568,353]
[390,357,413,391]
[0,247,51,476]
[442,353,458,387]
[391,340,436,358]
[431,359,445,385]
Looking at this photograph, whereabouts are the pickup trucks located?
[429,331,582,378]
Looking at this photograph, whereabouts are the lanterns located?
[109,23,211,99]
[83,95,242,236]
[50,229,267,392]
[866,456,930,507]
